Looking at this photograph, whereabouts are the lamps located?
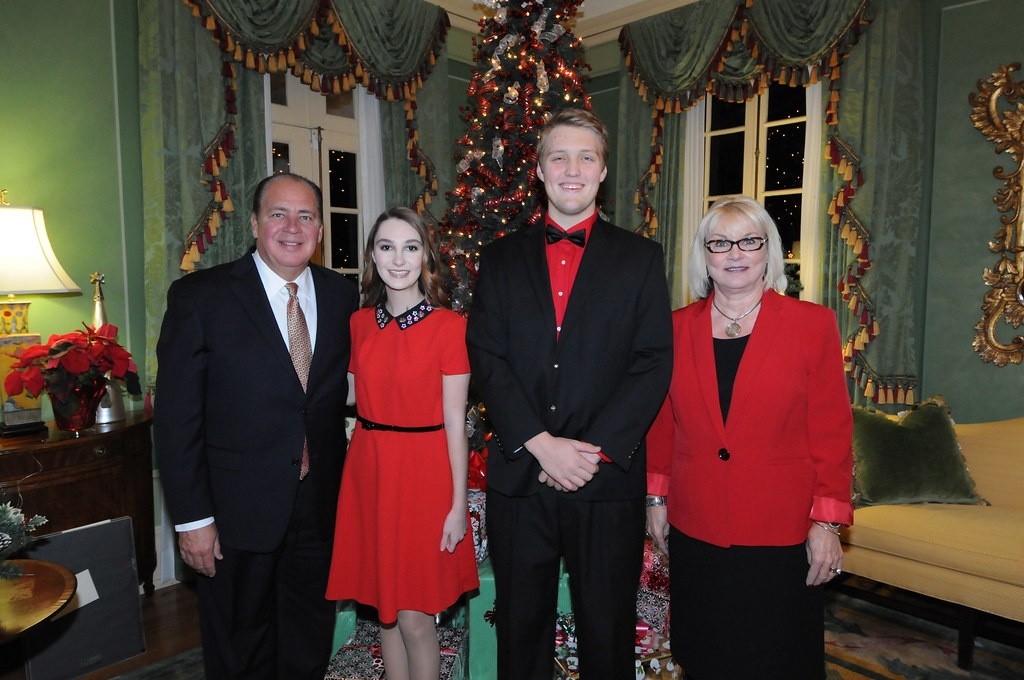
[0,188,82,429]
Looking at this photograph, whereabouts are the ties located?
[284,282,313,483]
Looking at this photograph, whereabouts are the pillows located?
[850,397,991,509]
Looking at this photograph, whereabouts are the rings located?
[830,567,842,574]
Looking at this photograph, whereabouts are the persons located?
[153,171,360,680]
[463,109,674,680]
[646,196,854,680]
[323,204,471,680]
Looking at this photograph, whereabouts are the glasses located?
[704,237,771,253]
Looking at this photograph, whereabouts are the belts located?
[356,414,445,431]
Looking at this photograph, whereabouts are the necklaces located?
[713,292,764,336]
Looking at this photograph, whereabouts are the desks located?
[0,408,160,596]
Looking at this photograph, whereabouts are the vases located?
[49,382,107,430]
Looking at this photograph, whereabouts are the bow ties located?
[545,223,587,248]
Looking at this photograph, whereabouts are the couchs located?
[835,414,1024,666]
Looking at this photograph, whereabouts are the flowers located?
[4,321,143,399]
[0,501,48,564]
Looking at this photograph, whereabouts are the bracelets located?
[644,530,649,537]
[812,520,842,536]
[644,496,667,508]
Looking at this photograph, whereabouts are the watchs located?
[825,522,842,529]
